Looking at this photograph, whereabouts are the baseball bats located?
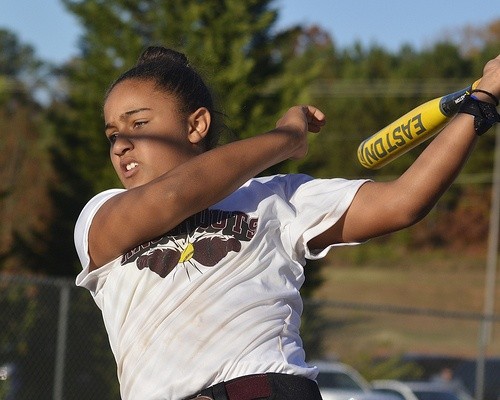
[355,77,482,171]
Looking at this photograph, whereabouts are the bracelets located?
[458,90,500,136]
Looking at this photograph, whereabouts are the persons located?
[73,47,500,399]
